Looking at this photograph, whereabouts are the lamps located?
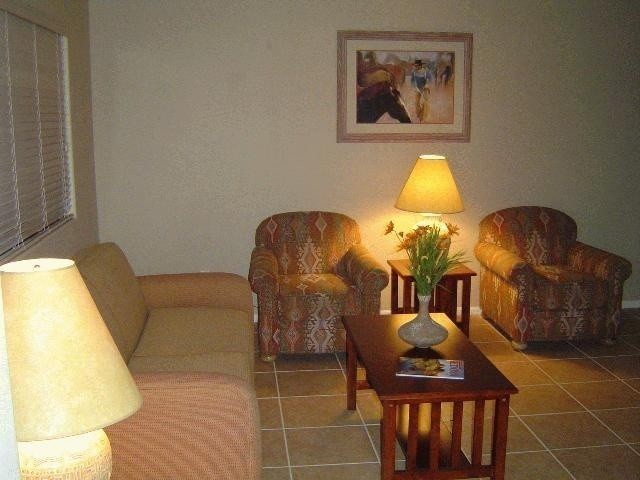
[394,153,466,230]
[0,257,144,480]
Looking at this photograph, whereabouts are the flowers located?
[384,220,472,296]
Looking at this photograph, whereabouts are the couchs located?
[76,240,263,480]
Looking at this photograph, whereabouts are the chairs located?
[474,206,632,351]
[248,210,390,362]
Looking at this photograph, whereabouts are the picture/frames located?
[336,28,474,144]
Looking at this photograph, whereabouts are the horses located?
[356,50,455,123]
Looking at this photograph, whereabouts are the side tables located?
[386,258,477,340]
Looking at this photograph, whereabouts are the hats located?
[413,59,425,66]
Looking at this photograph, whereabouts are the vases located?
[398,292,449,350]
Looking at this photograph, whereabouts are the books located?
[394,356,467,382]
